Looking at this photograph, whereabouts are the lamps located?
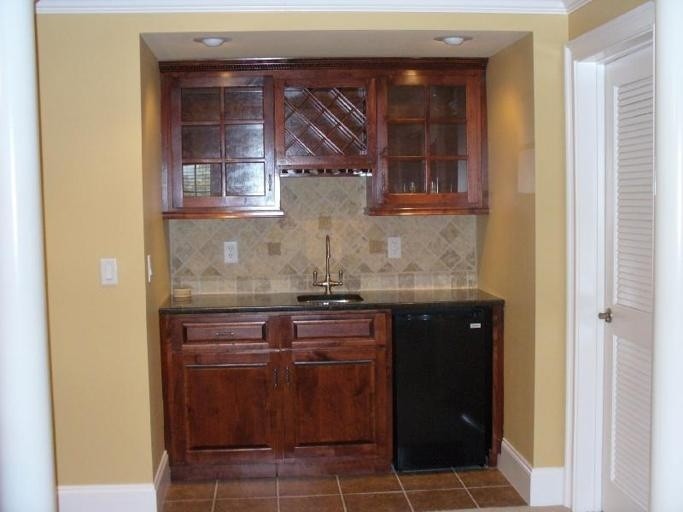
[433,36,474,45]
[192,37,229,48]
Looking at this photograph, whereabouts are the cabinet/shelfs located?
[157,309,393,481]
[160,70,286,219]
[367,69,490,216]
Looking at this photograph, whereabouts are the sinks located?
[297,294,364,304]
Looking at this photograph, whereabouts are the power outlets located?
[388,237,401,260]
[223,241,238,263]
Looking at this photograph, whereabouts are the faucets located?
[325,234,331,283]
